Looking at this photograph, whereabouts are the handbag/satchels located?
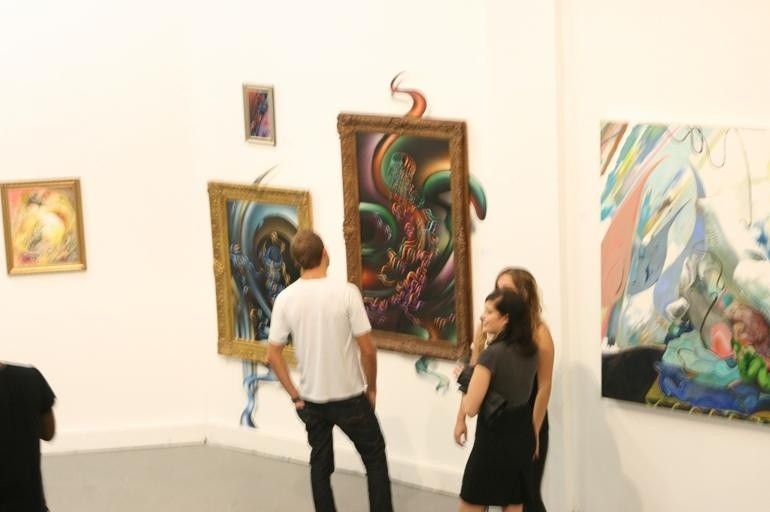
[457,366,506,430]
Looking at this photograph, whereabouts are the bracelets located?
[292,396,302,403]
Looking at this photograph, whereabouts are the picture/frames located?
[241,82,277,148]
[207,181,314,370]
[335,108,472,360]
[0,179,88,275]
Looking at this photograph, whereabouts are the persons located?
[0,362,56,512]
[266,230,392,512]
[455,268,554,511]
[453,289,539,510]
